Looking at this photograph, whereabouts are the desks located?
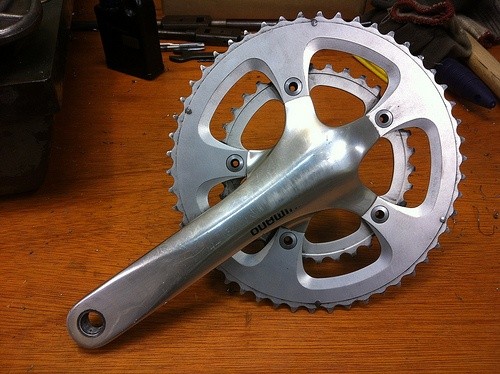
[2,24,500,374]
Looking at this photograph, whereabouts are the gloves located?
[373,3,492,68]
